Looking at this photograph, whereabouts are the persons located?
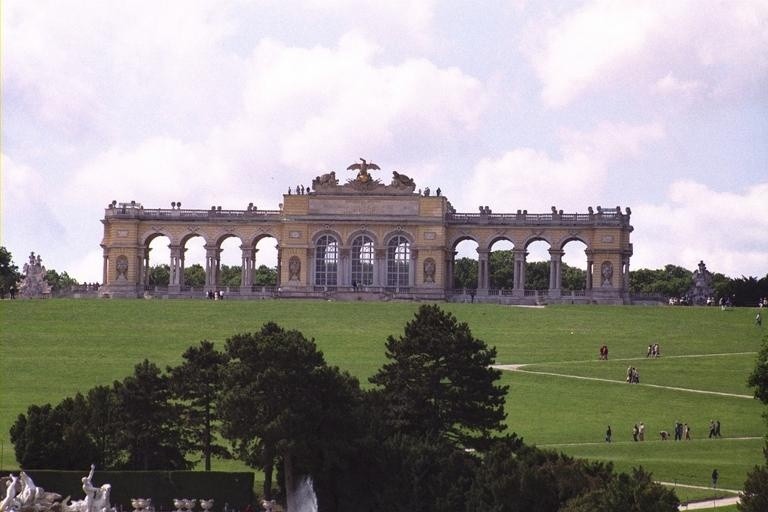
[632,419,724,442]
[759,297,764,310]
[352,281,357,291]
[711,468,719,489]
[10,284,15,301]
[603,345,608,361]
[606,425,611,443]
[668,294,736,312]
[763,297,768,308]
[626,365,632,382]
[357,280,363,291]
[13,470,36,503]
[0,472,22,512]
[654,343,660,357]
[81,463,100,512]
[755,311,761,326]
[652,342,657,357]
[646,344,652,357]
[363,282,368,292]
[599,345,605,360]
[206,288,225,301]
[635,368,640,384]
[631,367,636,381]
